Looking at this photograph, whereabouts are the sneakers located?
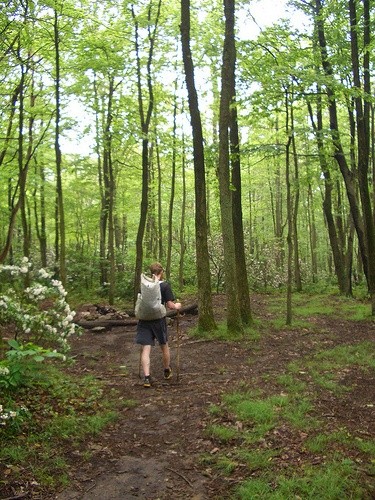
[164,369,172,379]
[144,379,152,387]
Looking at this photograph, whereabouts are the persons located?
[134,261,181,388]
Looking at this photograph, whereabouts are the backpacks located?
[134,273,167,320]
